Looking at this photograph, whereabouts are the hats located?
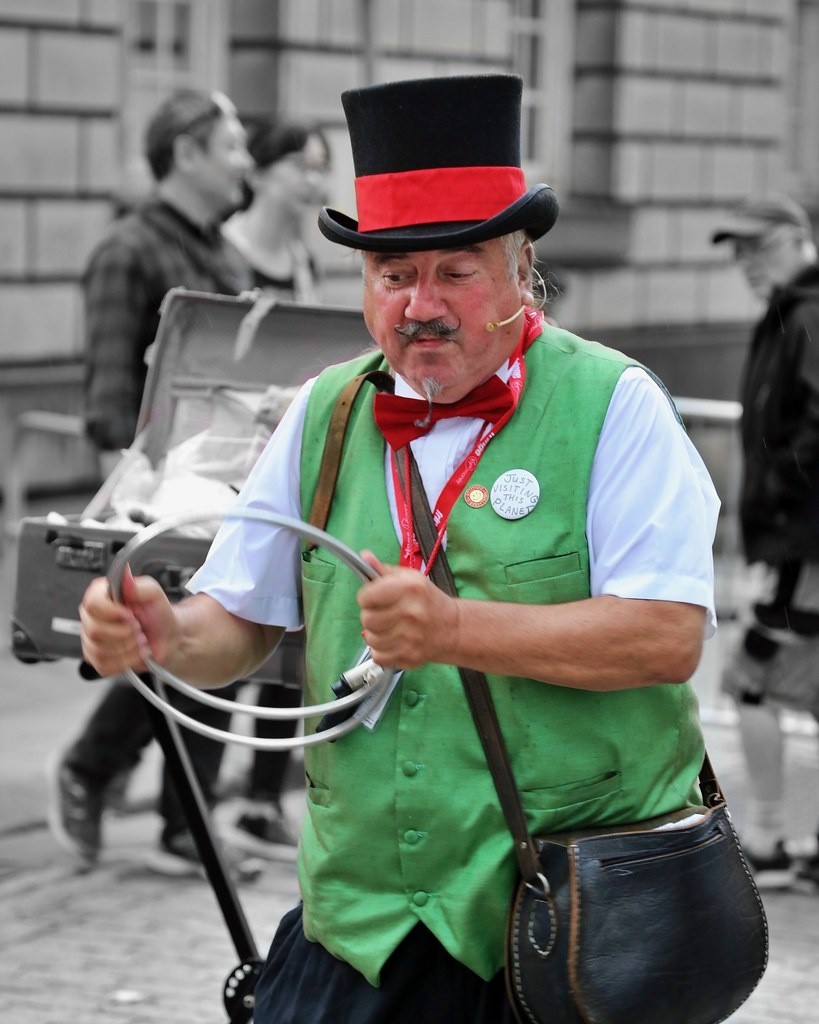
[710,192,812,246]
[317,73,559,252]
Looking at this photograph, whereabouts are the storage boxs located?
[10,283,378,689]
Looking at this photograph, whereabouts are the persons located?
[79,74,722,1024]
[710,192,819,895]
[47,89,334,884]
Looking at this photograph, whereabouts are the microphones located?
[486,305,526,333]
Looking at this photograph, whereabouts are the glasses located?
[181,89,236,134]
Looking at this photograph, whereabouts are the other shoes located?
[225,805,303,858]
[46,743,109,871]
[147,828,267,882]
[741,838,819,894]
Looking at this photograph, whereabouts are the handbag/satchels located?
[503,798,770,1024]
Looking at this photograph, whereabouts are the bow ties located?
[372,371,515,452]
